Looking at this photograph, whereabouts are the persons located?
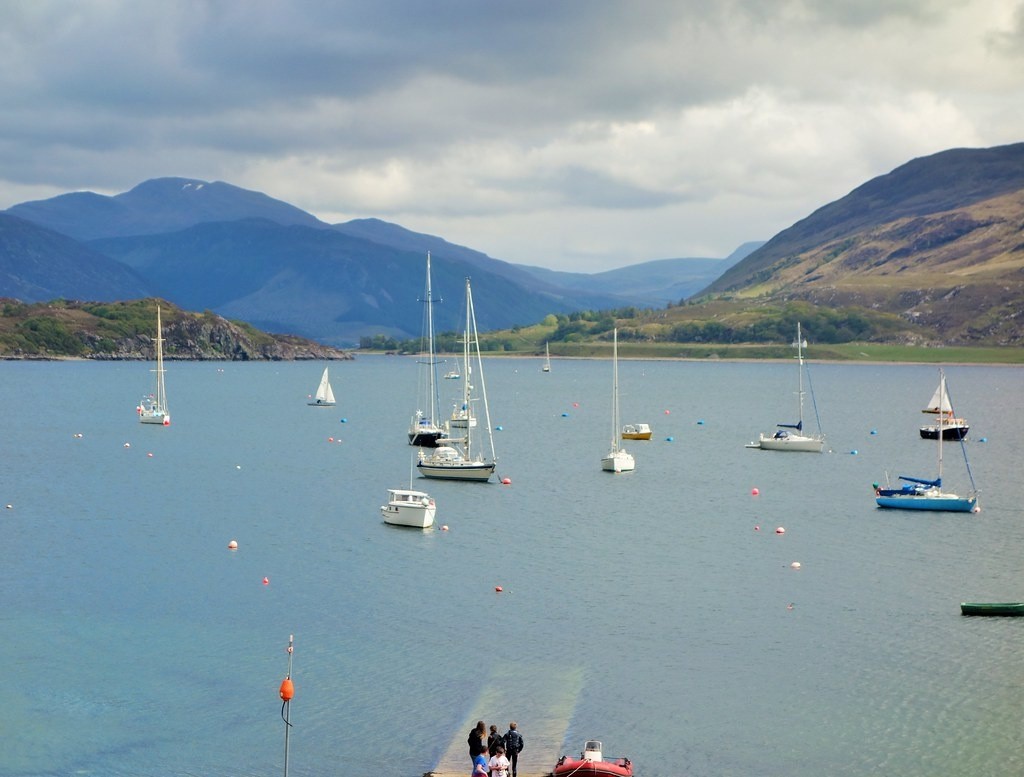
[488,725,502,758]
[503,722,523,777]
[472,746,489,777]
[468,721,486,762]
[488,748,510,777]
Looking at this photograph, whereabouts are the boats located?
[548,739,634,777]
[960,602,1024,617]
[621,424,653,440]
[380,451,436,529]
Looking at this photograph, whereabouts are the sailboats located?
[872,368,983,514]
[542,341,551,373]
[600,328,635,473]
[408,247,450,448]
[449,331,477,429]
[307,366,336,406]
[136,305,170,426]
[919,367,970,442]
[758,321,826,453]
[444,354,461,379]
[415,272,497,482]
